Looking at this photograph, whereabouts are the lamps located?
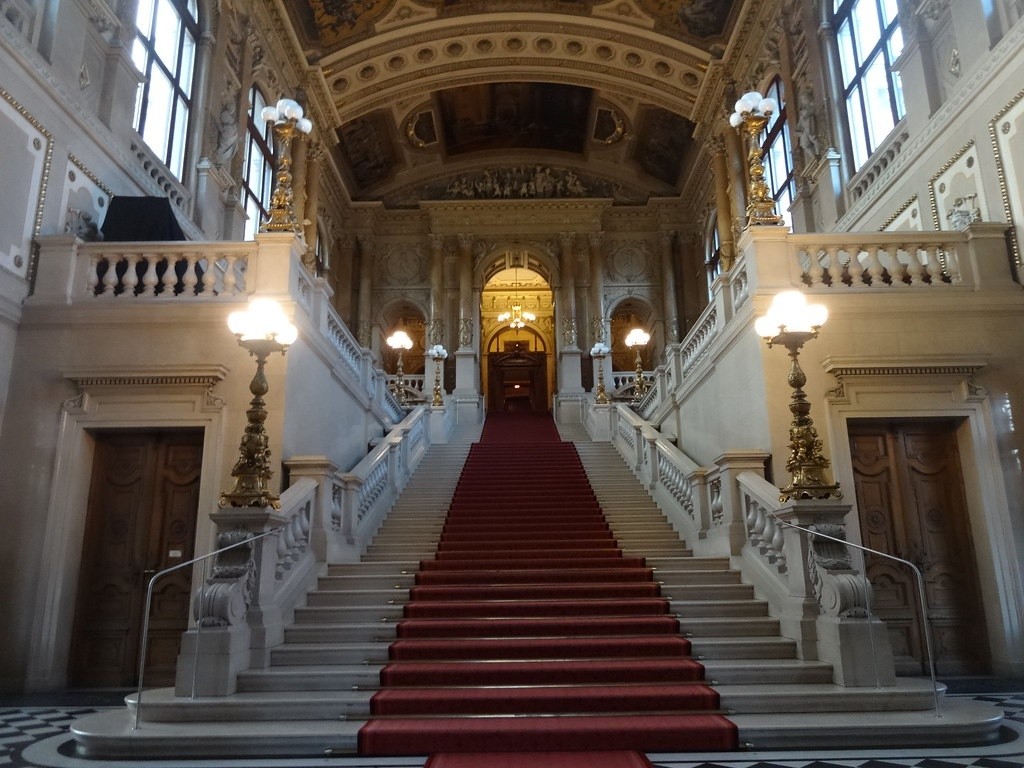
[428,345,448,406]
[217,296,298,512]
[387,331,413,406]
[730,91,785,231]
[259,99,312,239]
[498,269,536,336]
[625,328,651,404]
[753,290,843,503]
[589,343,615,404]
